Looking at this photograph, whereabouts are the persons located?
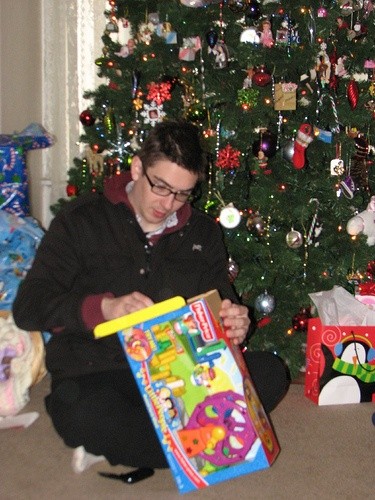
[11,122,294,484]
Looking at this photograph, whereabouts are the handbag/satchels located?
[304,316,375,407]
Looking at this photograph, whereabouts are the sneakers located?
[73,445,106,475]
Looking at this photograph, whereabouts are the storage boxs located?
[305,315,375,406]
[91,288,281,495]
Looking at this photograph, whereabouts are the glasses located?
[140,158,199,203]
